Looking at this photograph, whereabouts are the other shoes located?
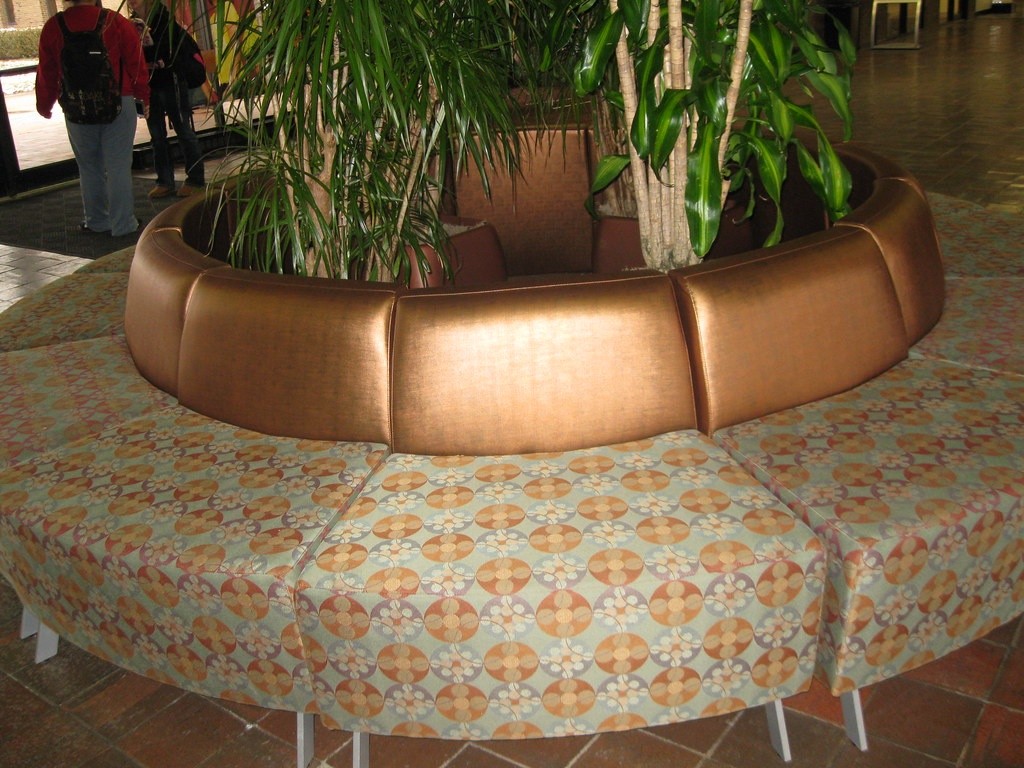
[149,185,204,197]
[79,219,143,231]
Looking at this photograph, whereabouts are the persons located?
[36,0,153,238]
[124,0,217,198]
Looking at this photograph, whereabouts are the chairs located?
[0,189,1024,768]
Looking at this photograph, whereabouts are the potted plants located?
[205,0,865,281]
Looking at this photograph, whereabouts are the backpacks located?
[170,19,207,90]
[53,9,123,124]
